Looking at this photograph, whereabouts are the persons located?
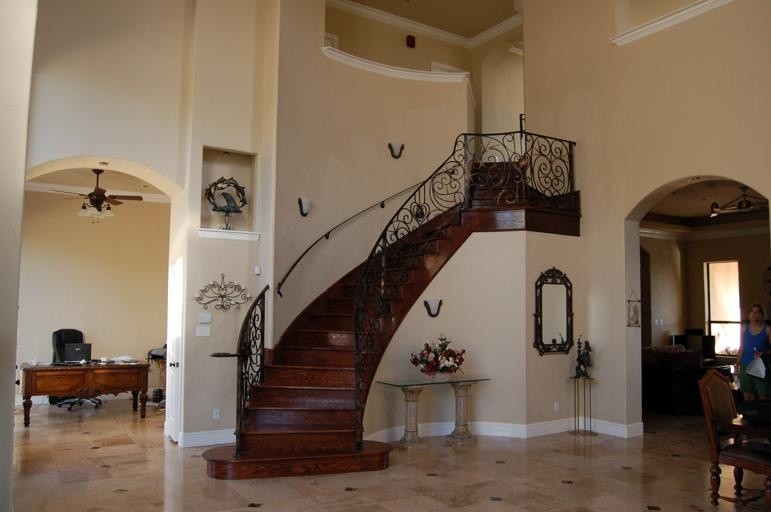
[733,304,770,424]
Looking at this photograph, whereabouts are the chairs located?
[49,328,103,411]
[698,368,771,508]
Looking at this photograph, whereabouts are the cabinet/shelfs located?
[673,333,716,368]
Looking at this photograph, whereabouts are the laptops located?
[51,343,91,366]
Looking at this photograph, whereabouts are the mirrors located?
[533,267,574,358]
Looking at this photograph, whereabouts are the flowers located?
[406,334,467,375]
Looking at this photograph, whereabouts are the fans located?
[49,169,143,212]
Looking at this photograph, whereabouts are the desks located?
[570,376,597,437]
[374,374,495,447]
[23,361,151,428]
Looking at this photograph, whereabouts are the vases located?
[433,370,451,381]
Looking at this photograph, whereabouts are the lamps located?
[709,185,768,220]
[385,142,407,160]
[77,197,115,221]
[192,272,252,313]
[296,195,312,219]
[423,298,445,319]
